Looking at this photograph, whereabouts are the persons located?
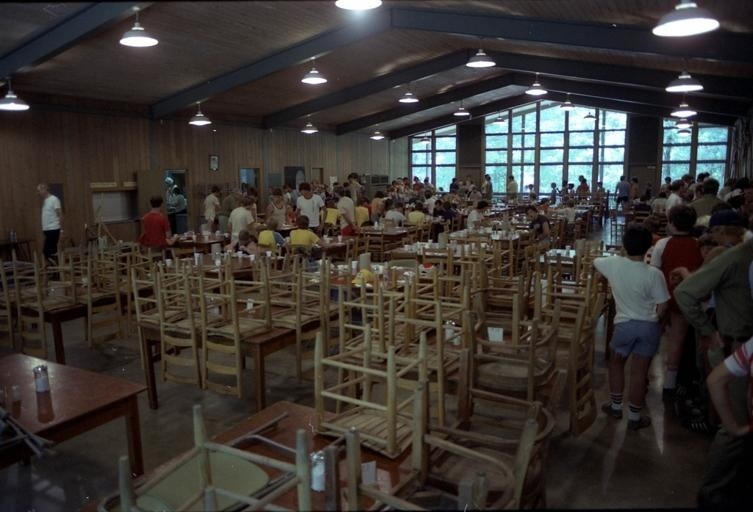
[204,186,221,224]
[167,188,187,221]
[644,181,653,200]
[559,180,568,201]
[614,176,631,213]
[347,172,363,204]
[581,179,588,192]
[529,192,538,206]
[551,183,556,205]
[409,202,425,225]
[680,174,692,184]
[652,193,667,209]
[651,205,704,415]
[434,177,467,218]
[223,230,256,256]
[660,177,671,193]
[165,178,177,199]
[258,216,285,255]
[741,183,753,223]
[643,216,661,247]
[139,196,179,253]
[652,209,668,234]
[311,180,325,204]
[294,182,328,238]
[667,180,684,214]
[265,188,288,228]
[593,225,672,431]
[506,175,518,193]
[707,335,753,446]
[701,210,743,265]
[287,215,325,268]
[528,184,533,192]
[568,183,576,194]
[227,197,260,245]
[686,179,726,216]
[595,181,605,196]
[524,206,550,256]
[483,174,493,199]
[694,185,705,199]
[576,175,589,192]
[355,198,369,227]
[467,175,480,200]
[467,201,488,229]
[385,202,408,228]
[36,183,65,281]
[538,198,548,215]
[333,186,354,228]
[374,176,434,202]
[673,236,753,512]
[551,199,575,232]
[630,176,640,200]
[724,175,749,200]
[697,173,714,183]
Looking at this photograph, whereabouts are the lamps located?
[583,111,596,121]
[665,71,704,94]
[368,126,384,140]
[119,10,159,46]
[526,73,548,96]
[453,102,469,115]
[301,117,319,134]
[492,113,508,126]
[301,61,327,85]
[650,1,720,38]
[467,48,495,68]
[560,93,575,110]
[397,85,419,103]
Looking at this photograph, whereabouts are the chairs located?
[338,283,397,418]
[119,405,310,512]
[274,256,325,386]
[132,262,196,367]
[536,279,600,432]
[348,388,539,511]
[78,255,130,347]
[200,263,272,396]
[316,325,428,459]
[1,253,49,348]
[16,253,78,358]
[158,265,233,384]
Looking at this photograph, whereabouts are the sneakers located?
[664,385,681,399]
[690,416,715,433]
[626,415,653,431]
[600,404,622,420]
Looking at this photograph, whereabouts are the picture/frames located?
[209,155,219,171]
